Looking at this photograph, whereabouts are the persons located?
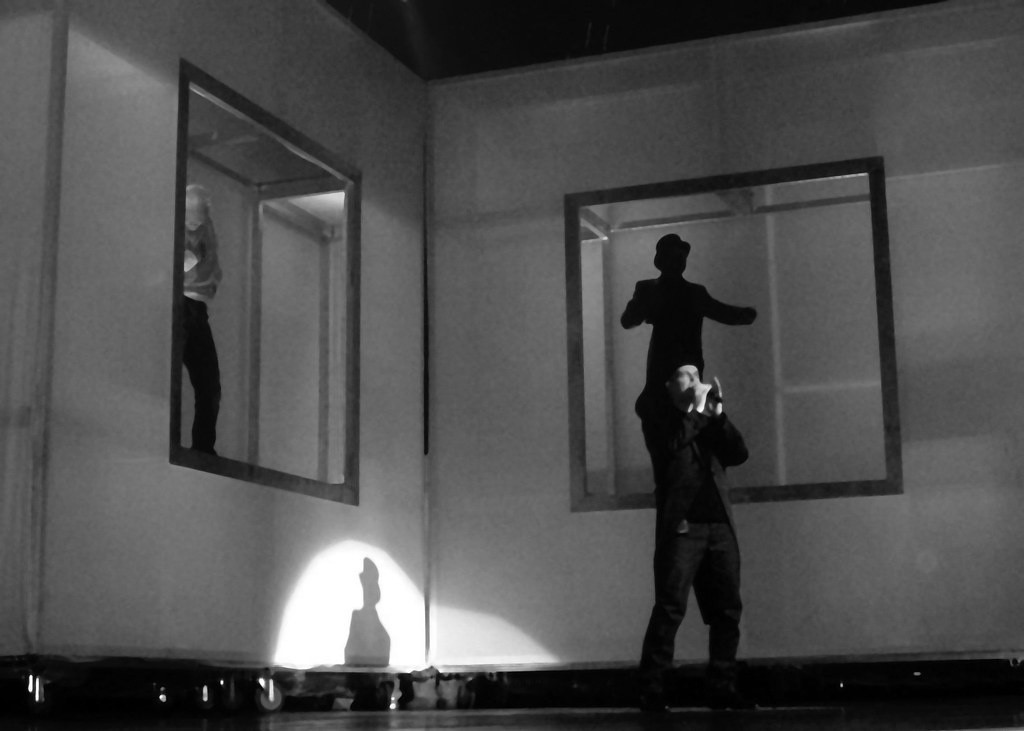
[620,229,759,388]
[633,334,761,716]
[181,183,225,458]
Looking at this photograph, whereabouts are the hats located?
[654,233,694,271]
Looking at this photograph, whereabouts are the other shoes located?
[636,687,674,717]
[707,678,760,714]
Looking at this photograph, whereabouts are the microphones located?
[706,393,723,402]
